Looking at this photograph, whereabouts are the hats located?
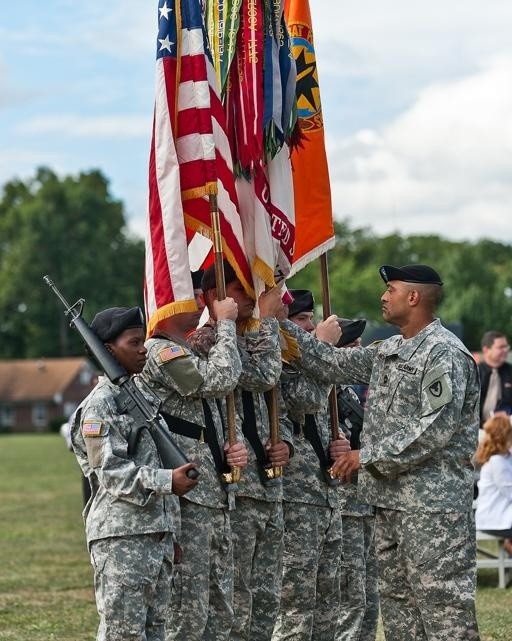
[201,257,235,292]
[191,269,204,289]
[379,264,444,286]
[83,307,144,351]
[289,291,314,317]
[335,317,366,348]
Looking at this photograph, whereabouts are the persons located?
[283,265,481,640]
[70,307,198,640]
[474,413,512,556]
[145,268,243,640]
[187,256,294,641]
[273,289,343,641]
[474,329,512,418]
[334,319,380,641]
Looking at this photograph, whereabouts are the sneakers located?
[499,541,512,555]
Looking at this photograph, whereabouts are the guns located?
[41,274,198,482]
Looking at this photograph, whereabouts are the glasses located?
[491,345,510,351]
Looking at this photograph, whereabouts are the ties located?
[483,368,502,423]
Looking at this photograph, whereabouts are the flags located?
[143,0,337,340]
